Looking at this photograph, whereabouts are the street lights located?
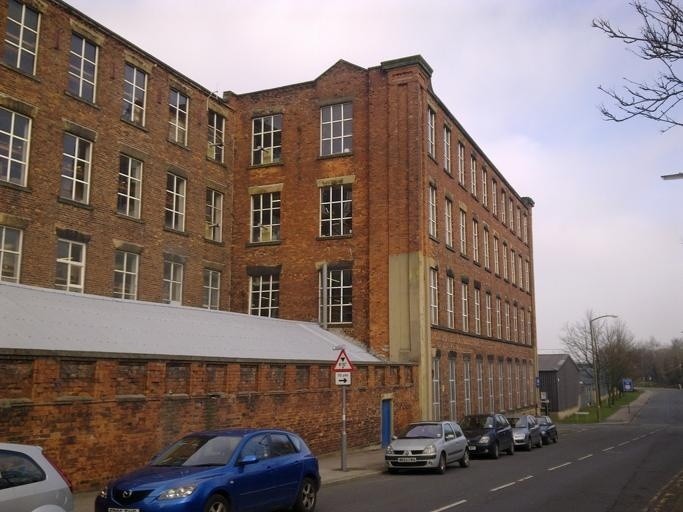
[590,314,619,416]
[660,171,683,182]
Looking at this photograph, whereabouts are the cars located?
[505,413,543,451]
[534,414,558,445]
[385,419,471,474]
[94,426,321,511]
[455,412,515,460]
[0,442,74,511]
